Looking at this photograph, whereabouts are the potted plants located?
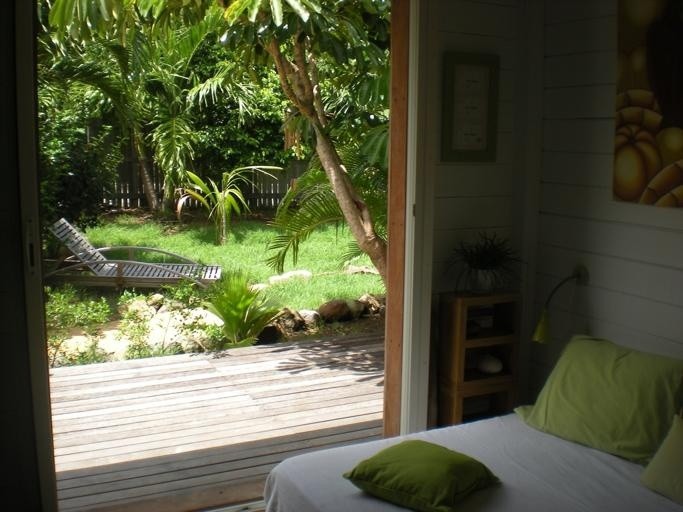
[440,229,509,296]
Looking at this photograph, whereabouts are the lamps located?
[531,265,587,344]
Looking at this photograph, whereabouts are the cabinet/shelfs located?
[433,286,524,426]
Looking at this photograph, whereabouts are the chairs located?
[42,216,222,292]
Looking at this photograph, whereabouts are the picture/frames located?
[439,50,500,163]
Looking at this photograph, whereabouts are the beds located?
[269,400,683,512]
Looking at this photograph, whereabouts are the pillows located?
[342,439,502,512]
[512,333,683,503]
